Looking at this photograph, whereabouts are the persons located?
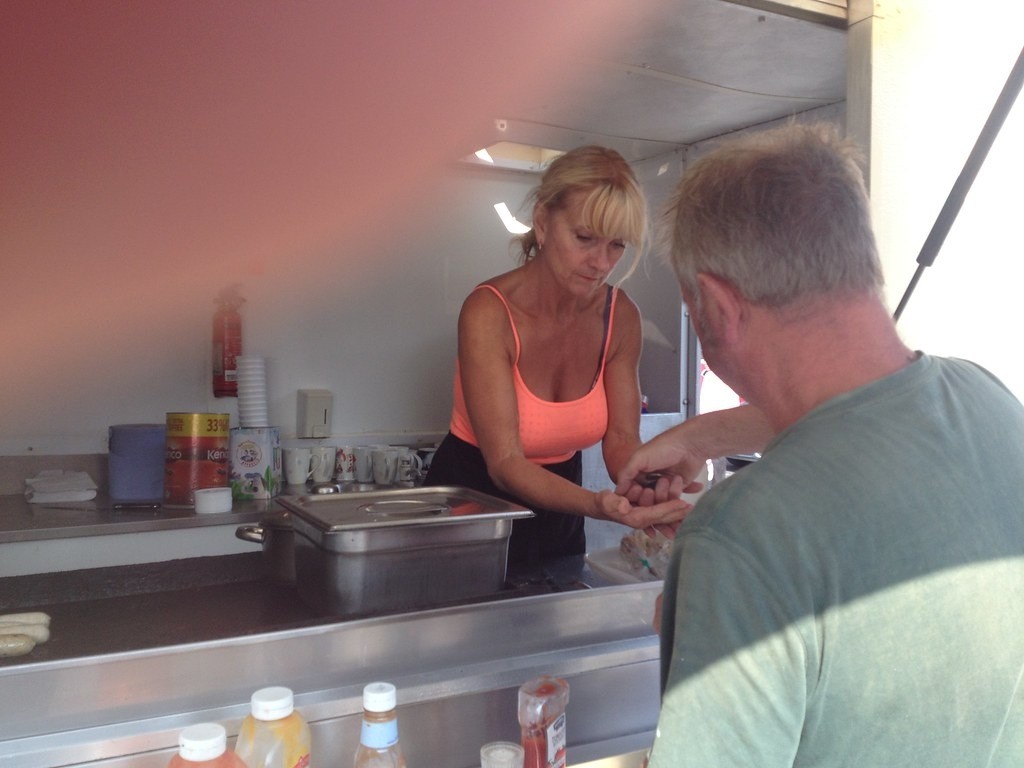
[616,121,1024,767]
[422,143,705,553]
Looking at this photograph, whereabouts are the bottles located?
[480,742,524,768]
[354,683,409,768]
[234,687,313,768]
[168,722,247,768]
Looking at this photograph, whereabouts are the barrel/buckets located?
[109,425,166,499]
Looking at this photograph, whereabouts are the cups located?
[388,446,414,482]
[355,447,374,482]
[337,446,354,481]
[283,447,320,484]
[235,355,268,428]
[410,450,422,472]
[311,446,335,482]
[420,448,437,474]
[373,450,398,485]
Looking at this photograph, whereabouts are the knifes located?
[114,502,194,512]
